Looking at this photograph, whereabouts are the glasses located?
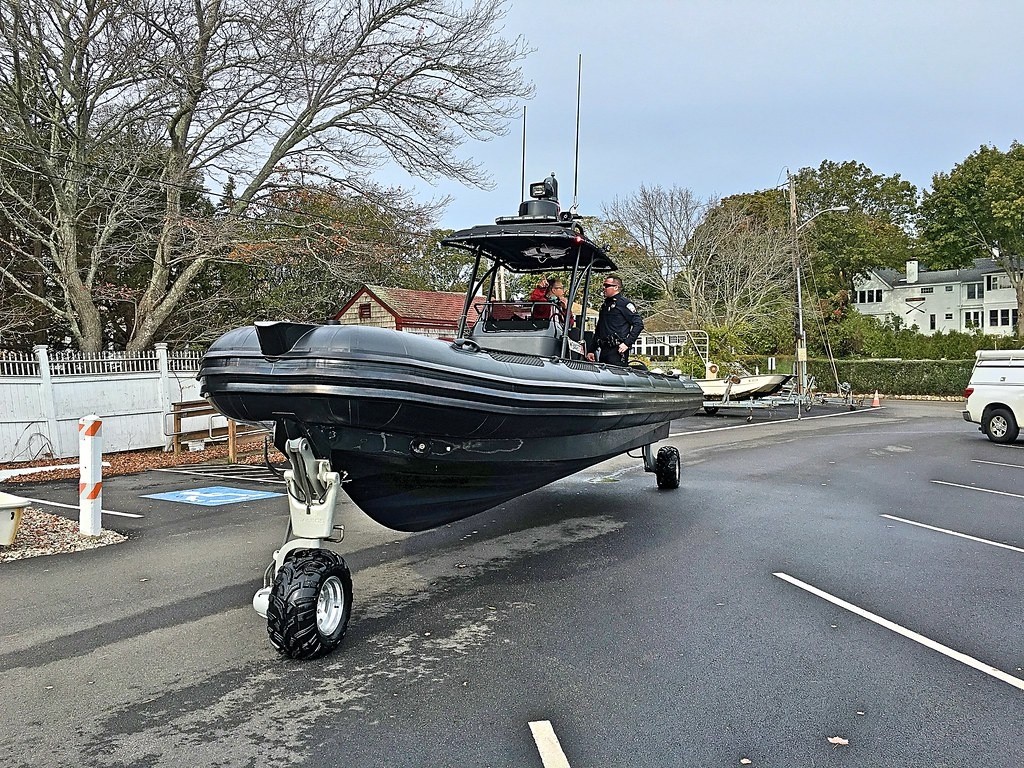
[554,286,564,289]
[603,283,617,288]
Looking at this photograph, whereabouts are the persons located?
[530,273,574,333]
[586,275,645,367]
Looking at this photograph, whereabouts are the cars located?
[963,349,1024,443]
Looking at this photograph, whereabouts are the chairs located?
[565,327,599,363]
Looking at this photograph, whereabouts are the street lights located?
[793,205,850,396]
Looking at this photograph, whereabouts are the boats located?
[680,368,797,400]
[194,52,703,534]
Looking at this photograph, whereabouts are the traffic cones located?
[871,390,883,408]
